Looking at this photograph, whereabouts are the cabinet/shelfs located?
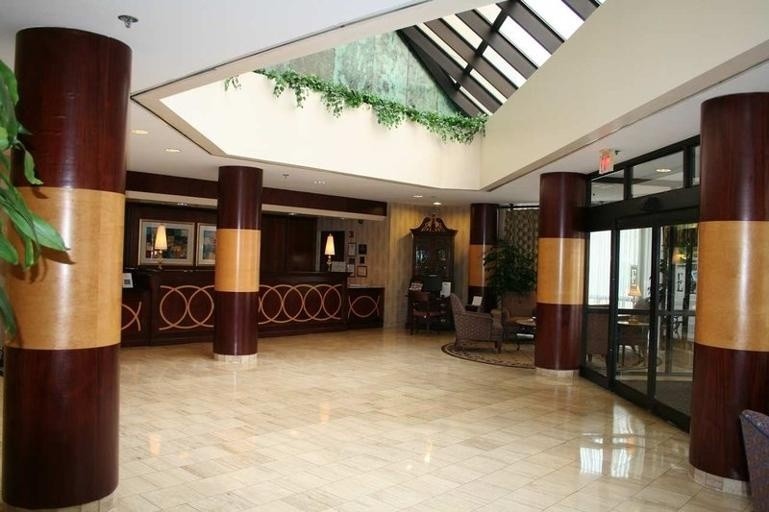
[404,214,459,298]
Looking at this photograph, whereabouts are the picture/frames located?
[137,216,217,271]
[121,266,138,293]
[345,241,369,278]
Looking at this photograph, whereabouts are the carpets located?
[440,340,533,370]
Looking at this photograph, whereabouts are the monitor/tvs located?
[123,268,136,292]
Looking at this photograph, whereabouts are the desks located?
[616,319,649,367]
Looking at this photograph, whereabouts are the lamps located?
[323,232,337,272]
[629,283,643,303]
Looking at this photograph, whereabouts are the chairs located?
[408,286,536,352]
